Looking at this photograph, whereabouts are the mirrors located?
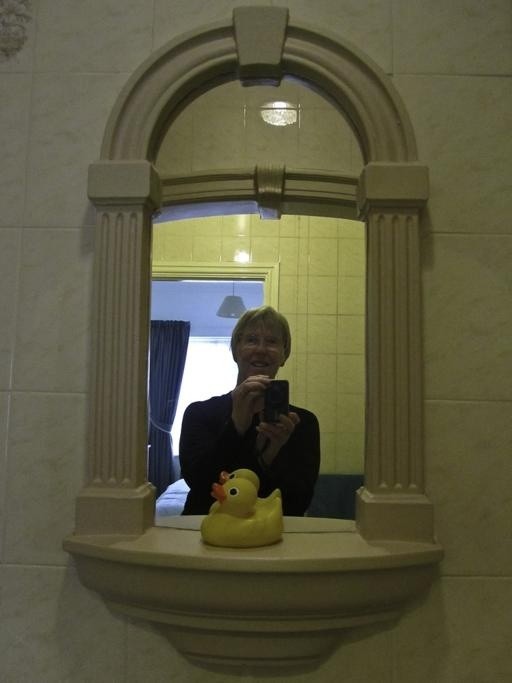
[145,198,369,532]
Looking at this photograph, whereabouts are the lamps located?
[217,283,246,317]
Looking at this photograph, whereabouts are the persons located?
[178,305,320,518]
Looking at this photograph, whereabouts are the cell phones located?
[264,379,290,424]
